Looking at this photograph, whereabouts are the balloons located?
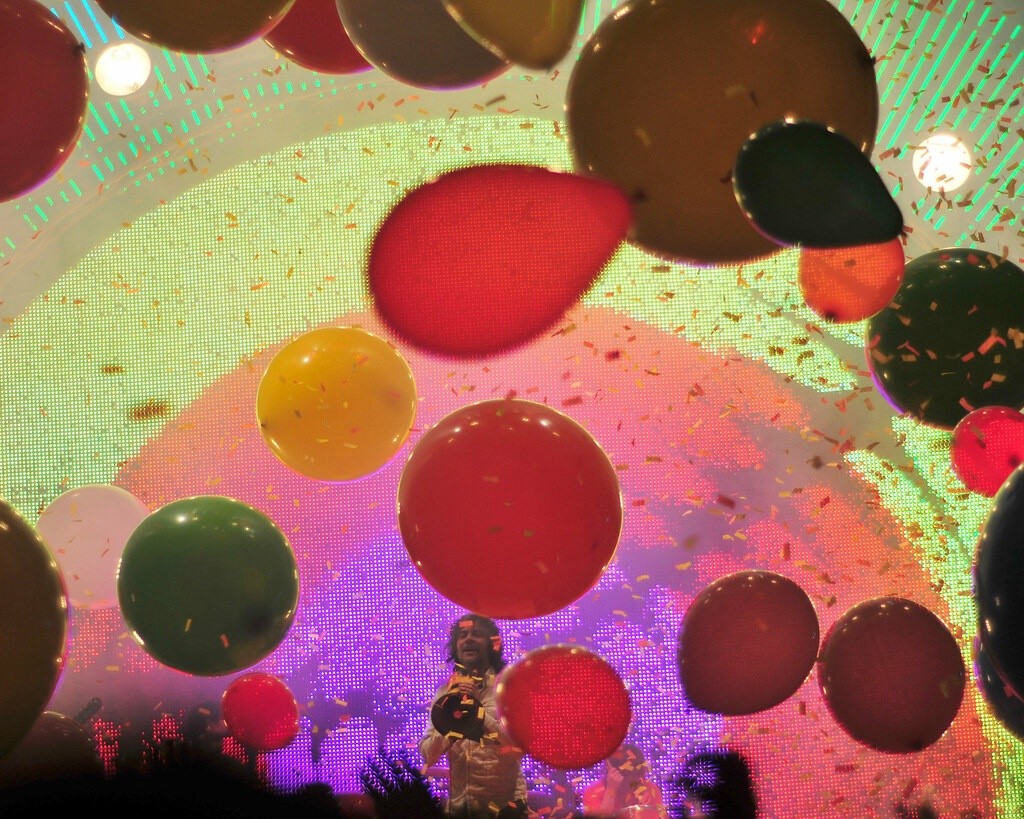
[0,1,1024,791]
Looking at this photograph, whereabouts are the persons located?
[419,615,528,818]
[584,744,666,819]
